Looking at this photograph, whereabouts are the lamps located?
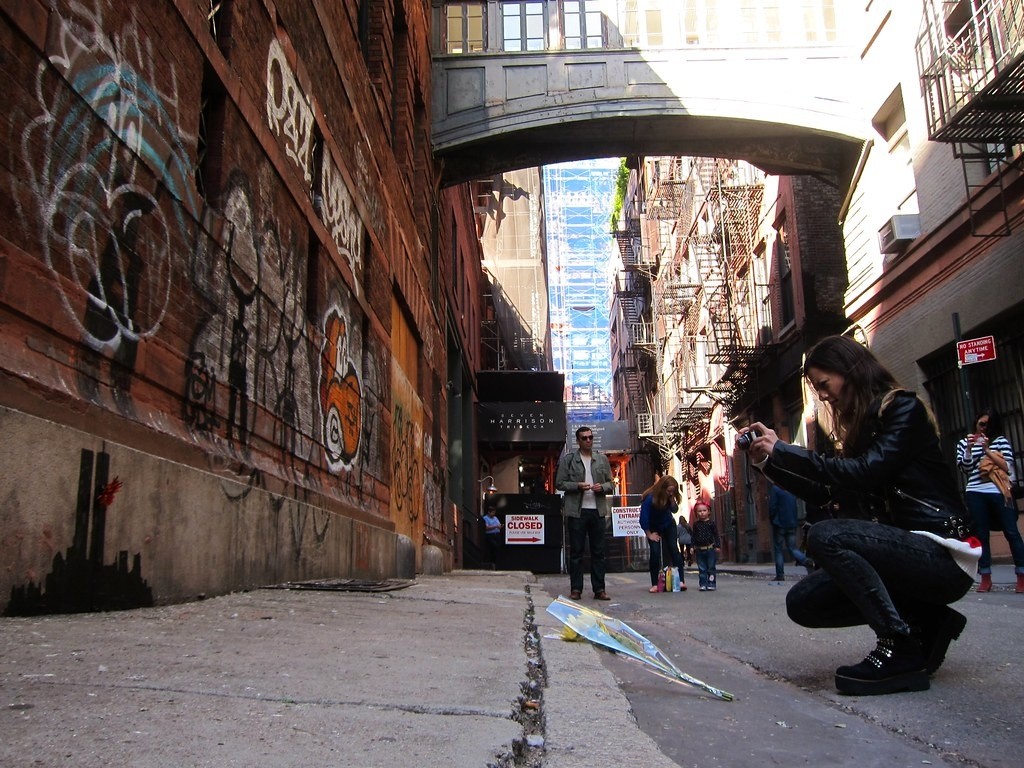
[477,476,498,495]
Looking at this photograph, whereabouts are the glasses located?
[581,435,594,441]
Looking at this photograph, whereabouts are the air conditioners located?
[876,213,922,255]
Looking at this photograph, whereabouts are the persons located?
[639,474,688,593]
[483,506,504,570]
[689,502,721,591]
[957,407,1024,593]
[556,427,615,602]
[677,516,693,566]
[730,334,984,693]
[768,484,815,581]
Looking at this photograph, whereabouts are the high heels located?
[1015,574,1024,592]
[835,630,930,695]
[976,574,992,592]
[912,605,967,674]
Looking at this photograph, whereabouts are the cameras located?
[737,429,757,450]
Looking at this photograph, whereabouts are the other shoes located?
[707,587,716,589]
[680,582,687,589]
[772,577,784,581]
[650,586,657,592]
[699,586,706,590]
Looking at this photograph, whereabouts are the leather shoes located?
[570,592,581,599]
[594,592,611,599]
